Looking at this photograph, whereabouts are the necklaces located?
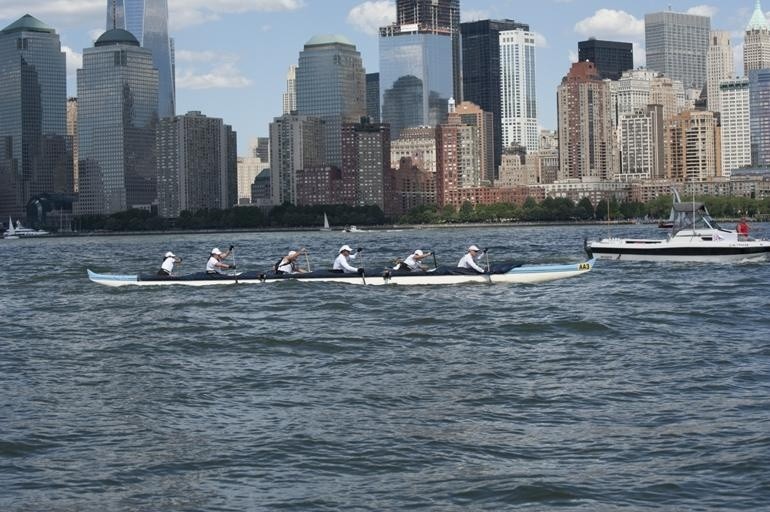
[320,212,332,232]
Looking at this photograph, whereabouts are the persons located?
[735,218,751,241]
[272,247,313,274]
[157,251,183,276]
[205,244,236,275]
[458,244,489,273]
[394,248,439,272]
[332,244,364,275]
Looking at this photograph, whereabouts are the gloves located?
[584,167,770,264]
[0,213,51,240]
[342,224,403,233]
[86,255,598,287]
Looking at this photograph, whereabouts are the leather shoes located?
[357,248,362,252]
[358,268,364,273]
[229,245,234,251]
[229,265,236,269]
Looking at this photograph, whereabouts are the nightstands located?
[486,251,494,284]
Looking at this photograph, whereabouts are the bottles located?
[468,245,480,251]
[211,248,222,255]
[414,248,424,256]
[165,251,175,257]
[288,250,297,256]
[338,244,353,253]
